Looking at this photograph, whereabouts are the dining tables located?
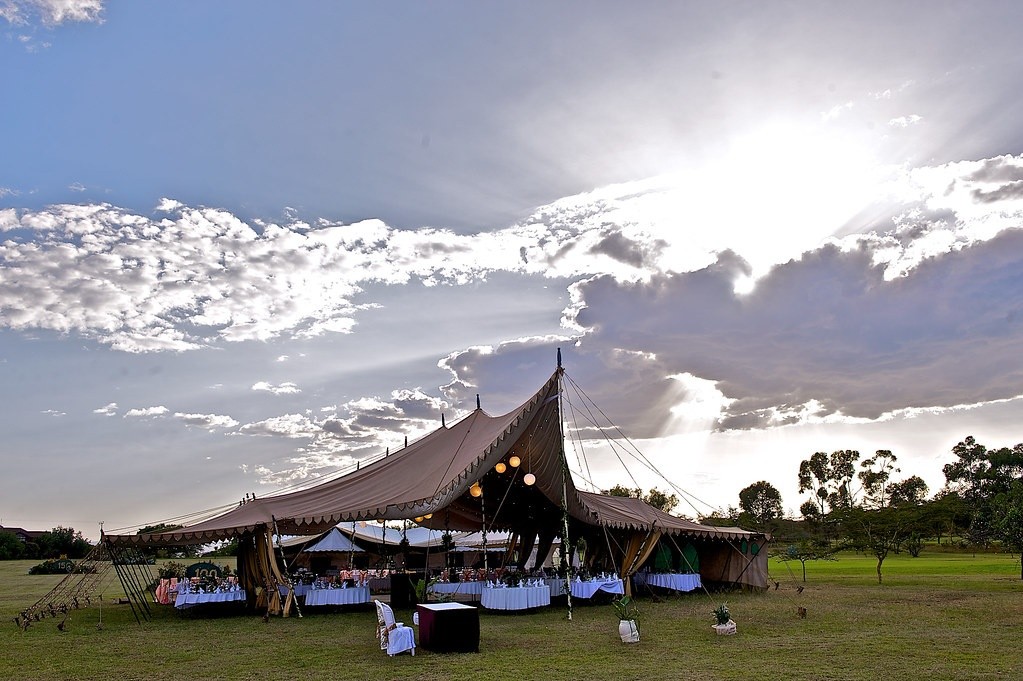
[155,564,705,610]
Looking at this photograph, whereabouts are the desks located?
[417,602,482,654]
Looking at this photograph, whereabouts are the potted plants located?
[709,605,737,636]
[611,593,643,644]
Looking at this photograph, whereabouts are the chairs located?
[380,600,416,658]
[373,597,405,656]
